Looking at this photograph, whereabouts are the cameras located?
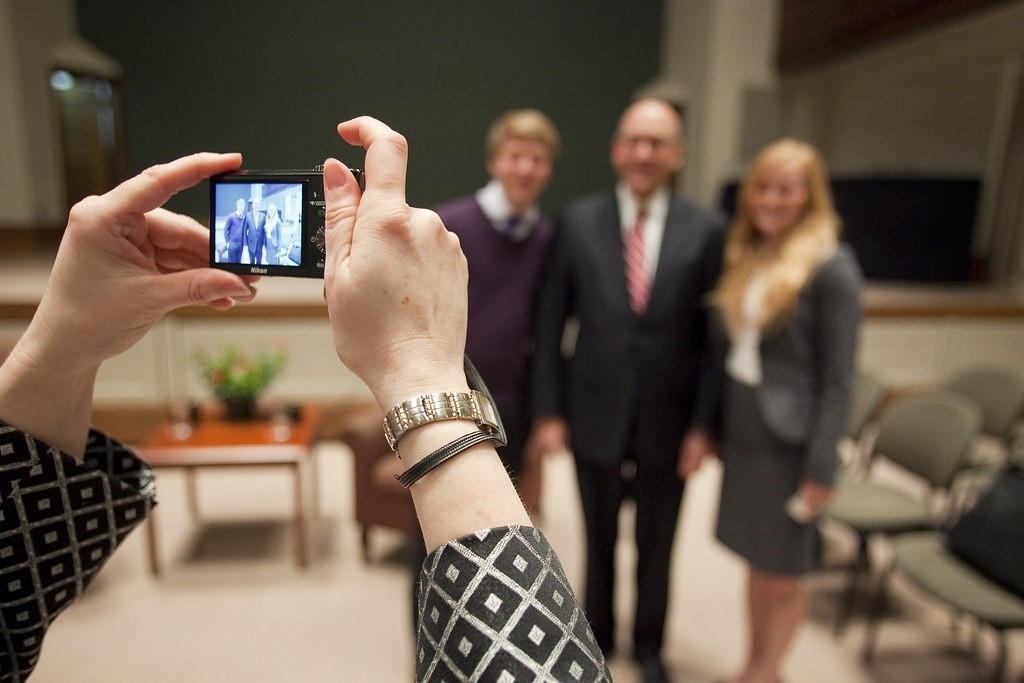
[210,164,366,278]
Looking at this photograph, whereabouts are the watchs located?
[381,389,506,456]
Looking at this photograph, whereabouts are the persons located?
[410,104,585,637]
[702,136,863,683]
[534,98,728,683]
[222,198,287,265]
[0,116,612,683]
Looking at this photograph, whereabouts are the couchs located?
[337,406,546,565]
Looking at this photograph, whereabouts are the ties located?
[622,212,653,314]
[504,216,520,239]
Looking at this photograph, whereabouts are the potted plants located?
[191,337,288,422]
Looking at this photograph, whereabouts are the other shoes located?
[635,646,669,683]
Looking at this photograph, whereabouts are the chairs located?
[814,359,1024,683]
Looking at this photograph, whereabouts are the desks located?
[130,401,326,581]
